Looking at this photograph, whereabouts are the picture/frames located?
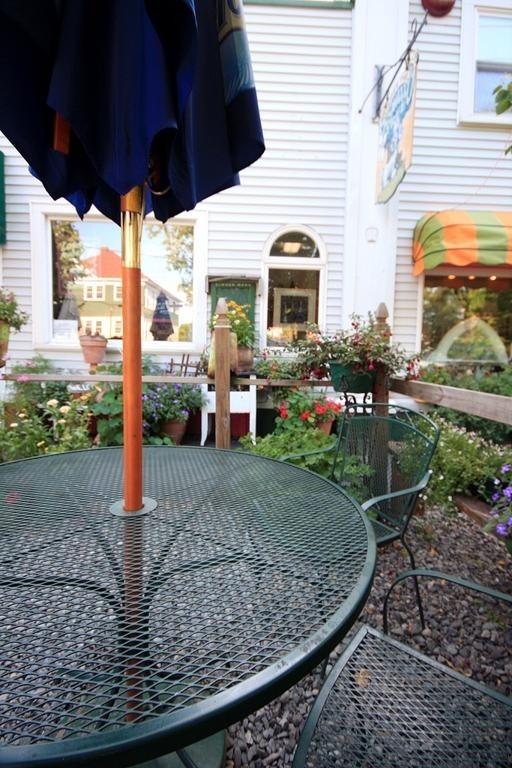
[28,205,204,356]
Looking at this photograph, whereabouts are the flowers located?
[141,381,208,421]
[0,288,31,333]
[291,312,420,382]
[208,301,254,345]
[276,396,342,420]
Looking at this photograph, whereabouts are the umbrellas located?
[149,289,174,341]
[0,0,267,726]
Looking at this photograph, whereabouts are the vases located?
[80,334,108,365]
[234,346,253,376]
[330,359,378,394]
[0,322,9,367]
